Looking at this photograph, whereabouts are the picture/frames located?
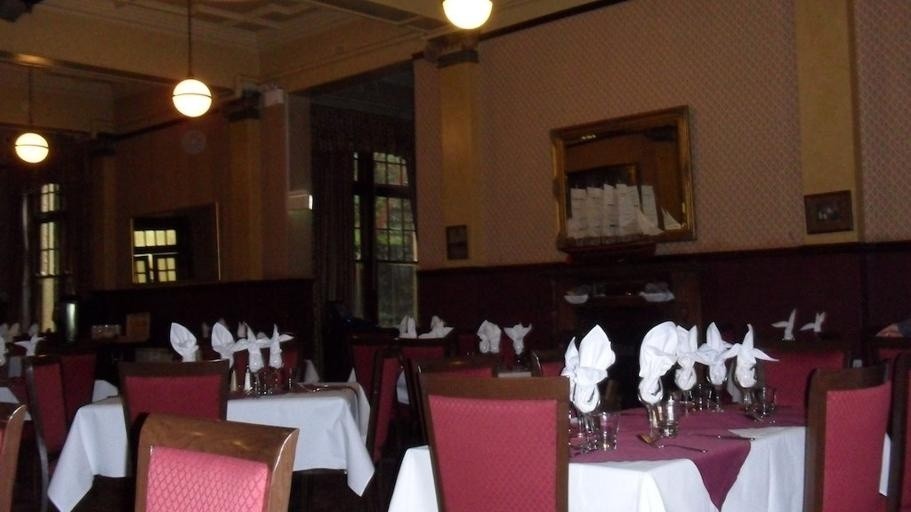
[444,223,468,262]
[802,189,855,235]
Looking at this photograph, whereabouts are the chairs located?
[536,361,579,417]
[753,348,845,415]
[801,369,892,512]
[116,360,229,424]
[20,355,94,512]
[56,352,95,421]
[133,411,298,512]
[301,355,399,512]
[878,355,911,512]
[459,333,515,369]
[864,336,910,372]
[416,372,568,512]
[395,337,446,446]
[0,404,28,512]
[232,349,300,395]
[342,336,411,422]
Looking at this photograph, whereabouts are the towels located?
[170,323,199,362]
[718,324,778,388]
[637,321,679,407]
[267,325,293,368]
[478,320,493,353]
[573,324,616,413]
[236,322,246,337]
[219,318,229,329]
[674,325,698,390]
[407,318,417,337]
[561,338,579,402]
[772,308,796,340]
[431,316,444,337]
[211,323,235,369]
[503,323,531,355]
[399,316,407,335]
[202,322,209,338]
[486,323,501,353]
[15,334,47,357]
[696,322,733,384]
[233,327,268,372]
[801,312,826,332]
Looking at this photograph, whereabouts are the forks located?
[639,432,708,454]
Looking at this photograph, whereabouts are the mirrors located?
[126,199,223,288]
[548,103,698,252]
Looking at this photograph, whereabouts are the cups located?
[749,385,777,425]
[706,384,725,413]
[268,366,285,388]
[565,406,595,458]
[512,355,525,371]
[682,384,705,417]
[249,369,265,397]
[809,327,827,348]
[741,387,754,417]
[637,391,682,440]
[592,397,622,454]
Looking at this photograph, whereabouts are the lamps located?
[170,0,212,119]
[11,66,50,164]
